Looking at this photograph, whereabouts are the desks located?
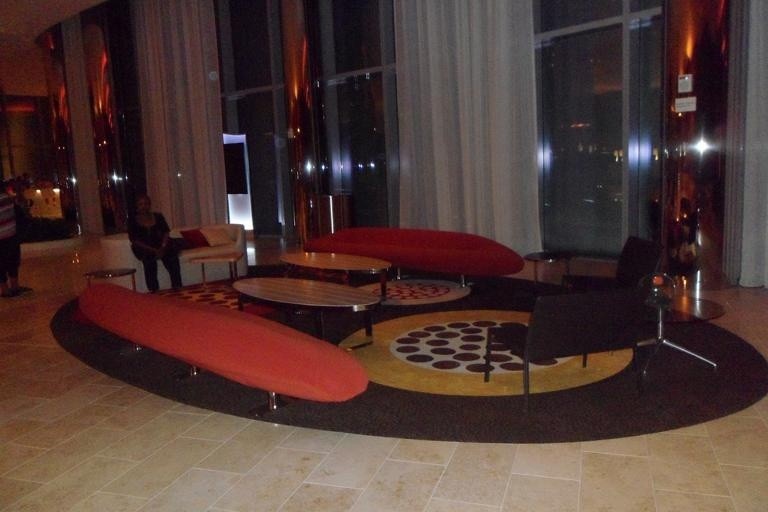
[84,269,136,292]
[634,298,725,379]
[233,254,391,353]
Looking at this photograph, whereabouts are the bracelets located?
[161,242,167,249]
[150,247,154,253]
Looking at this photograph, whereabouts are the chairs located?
[523,234,660,404]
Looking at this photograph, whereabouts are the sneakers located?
[172,281,182,289]
[2,285,32,299]
[151,286,159,292]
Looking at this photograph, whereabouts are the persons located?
[667,197,697,250]
[0,180,35,298]
[128,193,184,294]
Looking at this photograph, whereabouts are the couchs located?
[52,223,249,293]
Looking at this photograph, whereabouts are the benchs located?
[308,225,524,288]
[77,283,371,413]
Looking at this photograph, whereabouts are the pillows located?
[181,226,232,247]
